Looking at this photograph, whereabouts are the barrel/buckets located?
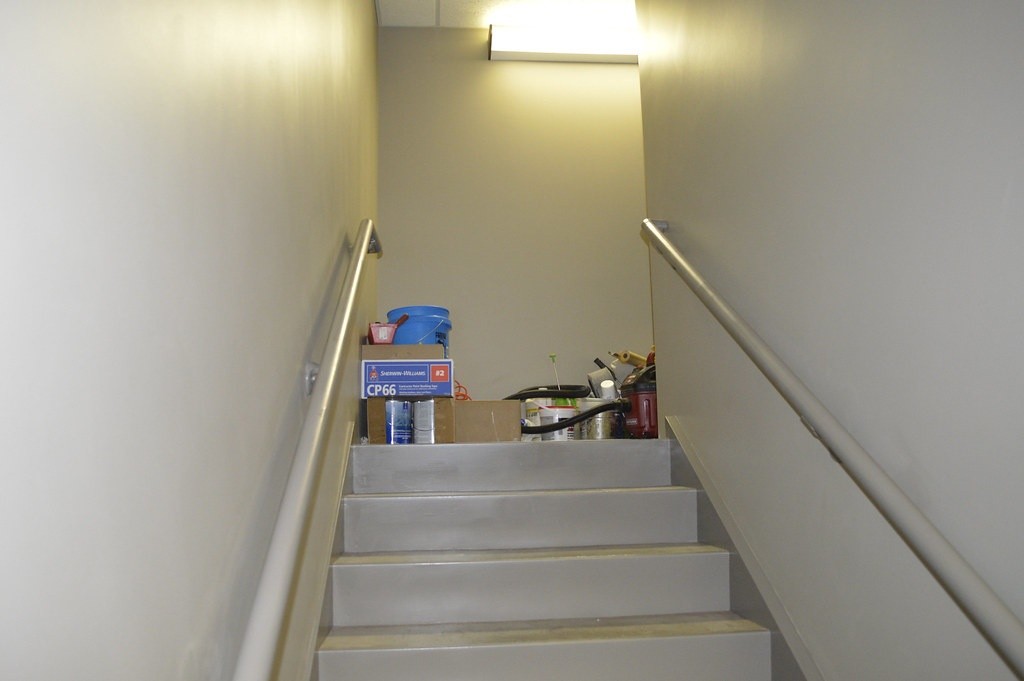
[524,381,658,441]
[386,305,452,359]
[384,398,435,443]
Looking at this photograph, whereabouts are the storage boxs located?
[360,343,521,443]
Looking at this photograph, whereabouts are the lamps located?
[487,24,638,63]
